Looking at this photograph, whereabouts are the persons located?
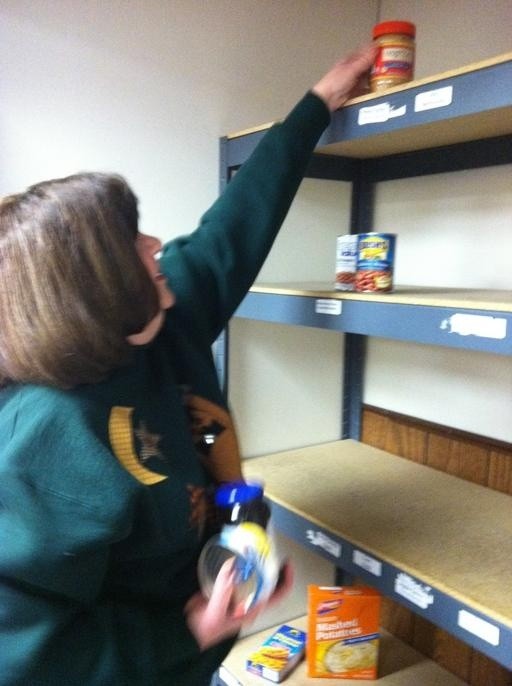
[0,47,380,686]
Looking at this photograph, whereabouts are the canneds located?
[333,234,358,291]
[354,232,396,294]
[368,21,416,93]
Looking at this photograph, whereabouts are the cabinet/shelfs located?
[214,50,512,685]
